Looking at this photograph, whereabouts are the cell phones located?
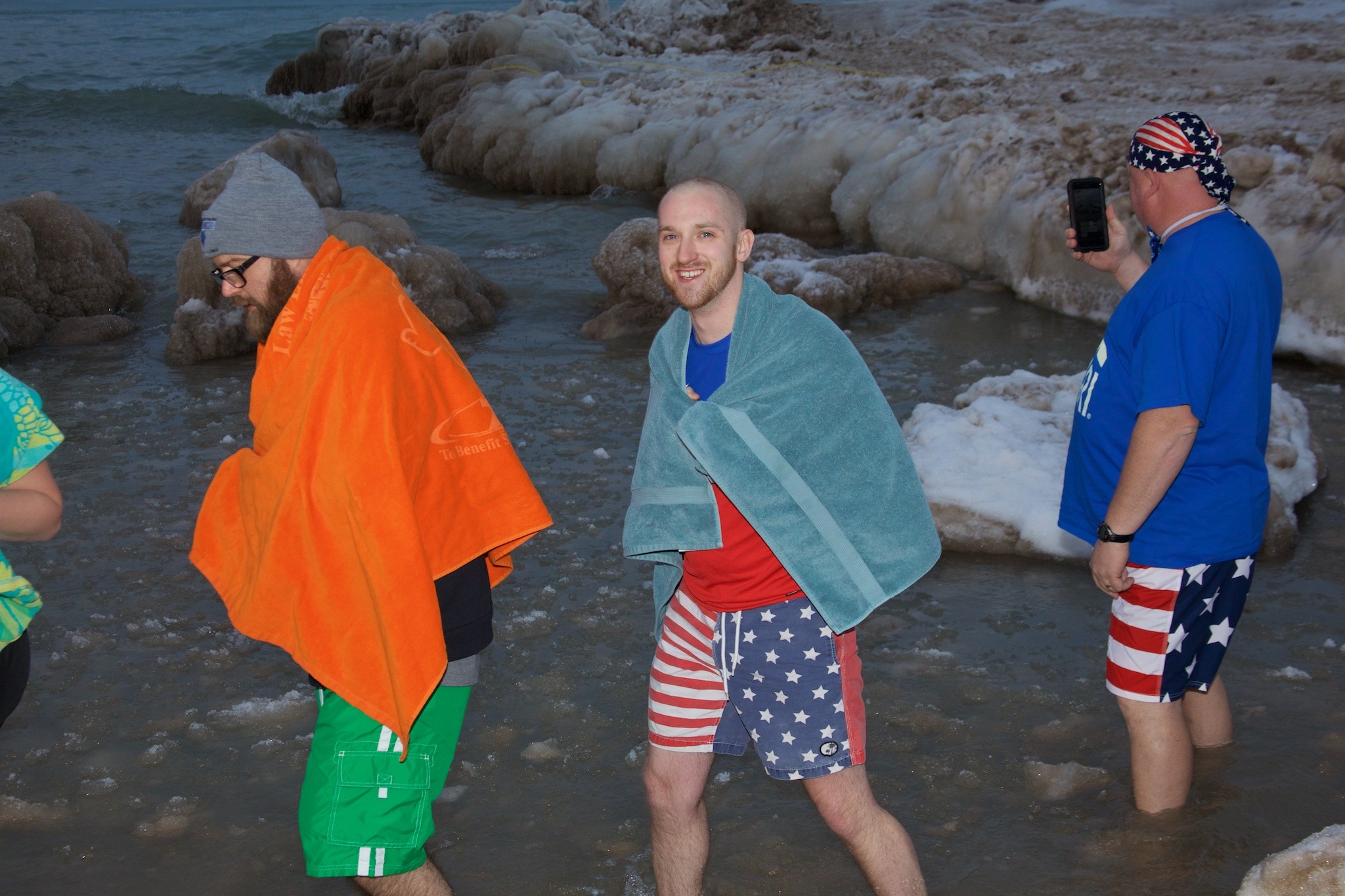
[1068,177,1109,253]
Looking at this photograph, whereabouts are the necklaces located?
[1148,205,1230,264]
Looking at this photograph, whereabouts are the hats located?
[200,152,327,260]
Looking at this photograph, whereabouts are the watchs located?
[1098,520,1135,543]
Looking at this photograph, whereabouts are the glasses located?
[209,255,260,289]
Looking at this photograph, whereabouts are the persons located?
[1,363,69,725]
[1058,111,1284,815]
[614,175,942,894]
[185,153,553,896]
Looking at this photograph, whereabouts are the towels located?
[620,273,942,638]
[190,232,557,765]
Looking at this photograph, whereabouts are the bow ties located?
[1147,204,1228,264]
